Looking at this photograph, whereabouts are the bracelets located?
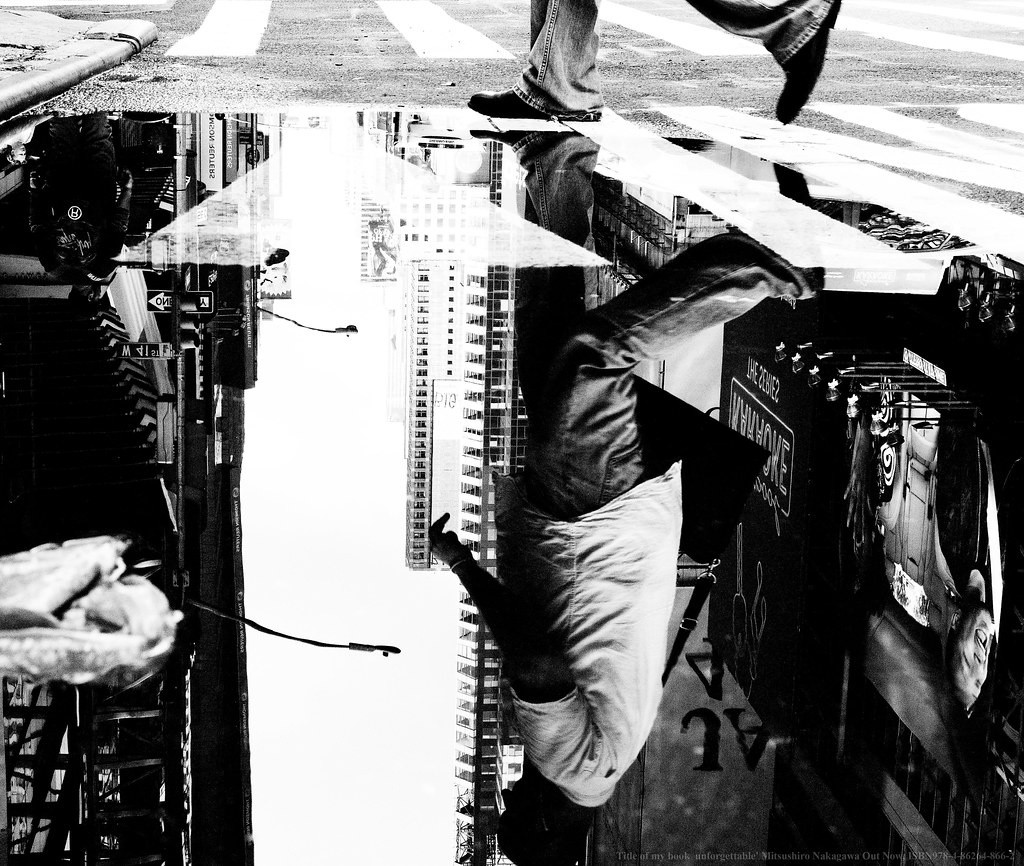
[449,556,477,571]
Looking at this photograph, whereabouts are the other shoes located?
[470,130,532,147]
[467,89,601,121]
[725,222,826,301]
[776,0,842,125]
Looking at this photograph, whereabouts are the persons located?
[3,112,52,168]
[927,407,1022,734]
[423,131,826,865]
[466,0,846,125]
[28,109,133,321]
